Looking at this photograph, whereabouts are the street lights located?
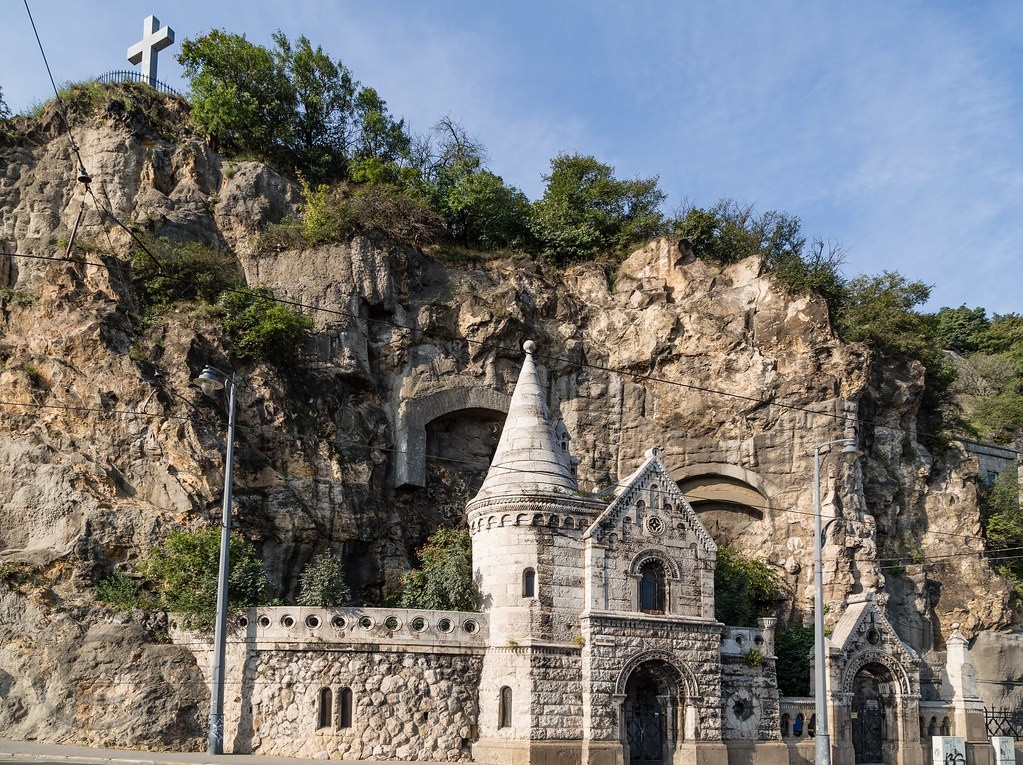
[193,364,240,755]
[815,437,865,765]
